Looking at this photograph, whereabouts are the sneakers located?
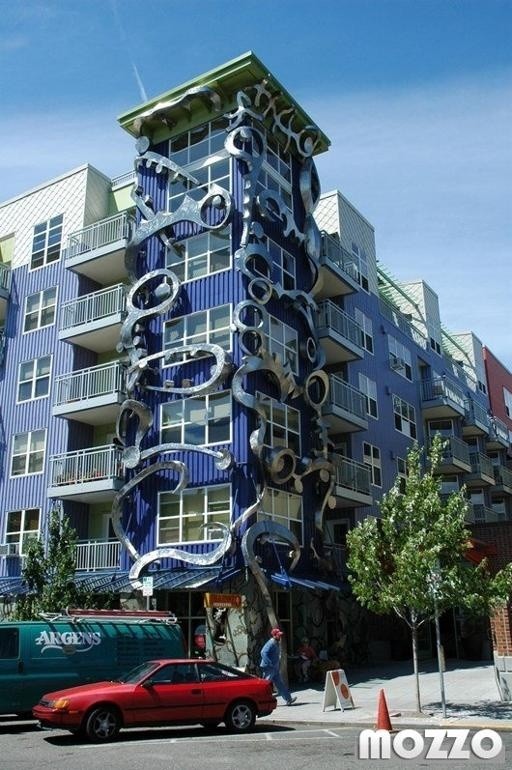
[287,696,297,705]
[297,675,302,681]
[304,677,310,682]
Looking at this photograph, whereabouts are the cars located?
[33,656,278,747]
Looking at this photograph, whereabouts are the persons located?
[259,628,297,706]
[295,638,321,683]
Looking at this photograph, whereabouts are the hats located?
[270,628,284,636]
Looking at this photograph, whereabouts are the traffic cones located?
[376,688,399,733]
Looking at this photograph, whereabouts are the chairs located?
[174,671,196,682]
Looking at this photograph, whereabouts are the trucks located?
[0,604,187,719]
